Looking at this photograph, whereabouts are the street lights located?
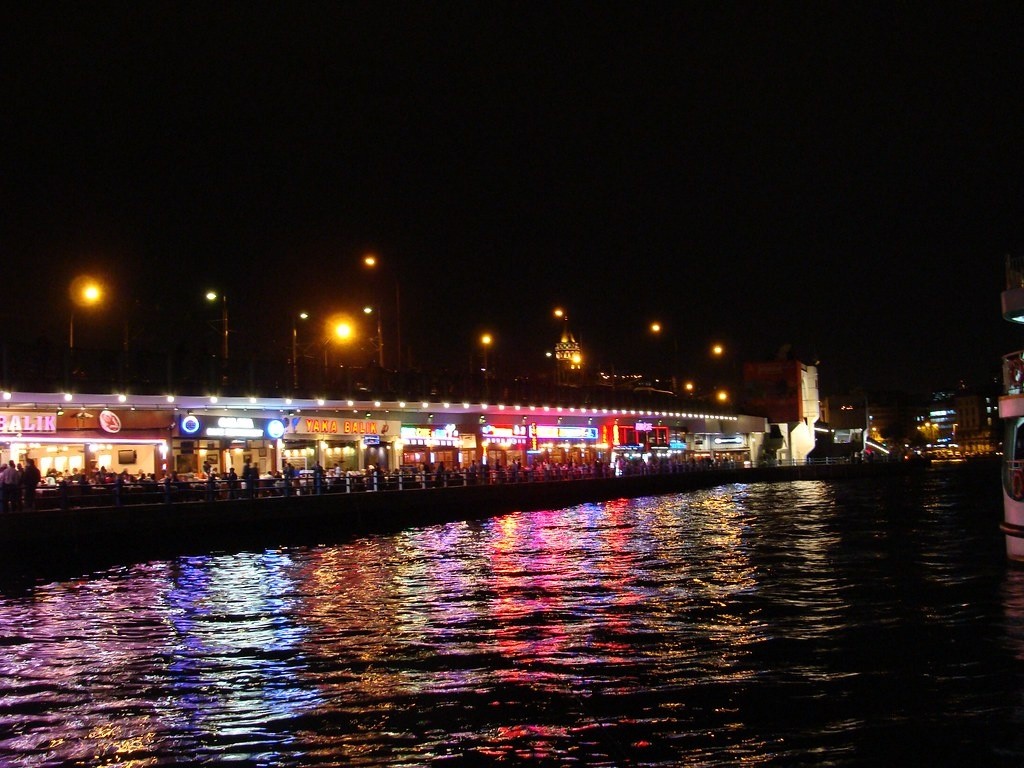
[361,303,389,369]
[288,310,314,397]
[204,287,233,394]
[59,273,106,351]
[360,254,403,368]
[480,335,495,388]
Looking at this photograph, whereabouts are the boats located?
[999,247,1023,564]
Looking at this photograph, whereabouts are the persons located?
[0,453,749,515]
